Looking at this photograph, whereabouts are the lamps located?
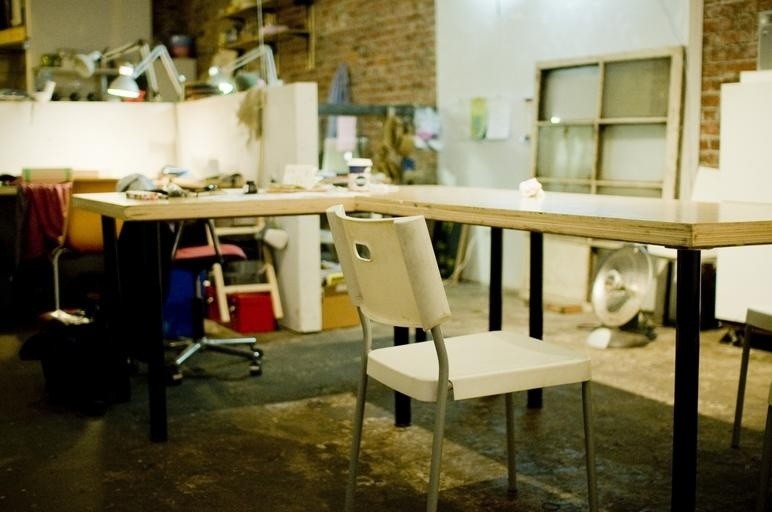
[74,33,284,99]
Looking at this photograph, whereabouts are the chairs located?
[325,203,598,508]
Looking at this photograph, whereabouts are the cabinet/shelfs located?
[223,0,319,78]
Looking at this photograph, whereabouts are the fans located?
[585,243,666,350]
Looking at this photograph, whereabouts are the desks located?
[60,181,771,508]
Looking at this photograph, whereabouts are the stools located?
[731,307,770,449]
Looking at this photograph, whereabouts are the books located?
[269,172,329,193]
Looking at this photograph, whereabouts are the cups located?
[347,157,373,192]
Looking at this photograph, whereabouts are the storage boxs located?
[323,275,361,331]
